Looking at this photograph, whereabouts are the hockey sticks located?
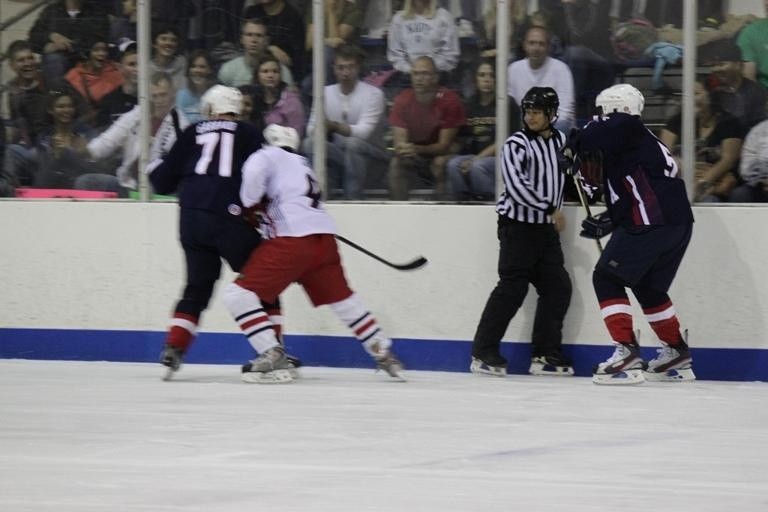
[336,233,427,270]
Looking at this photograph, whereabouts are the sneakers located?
[591,342,643,375]
[374,350,403,376]
[530,352,574,367]
[642,342,692,373]
[159,342,182,370]
[241,345,300,372]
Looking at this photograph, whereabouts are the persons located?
[1,2,768,203]
[558,84,695,375]
[146,84,299,370]
[471,87,601,367]
[221,124,390,372]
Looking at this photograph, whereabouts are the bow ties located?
[469,345,509,368]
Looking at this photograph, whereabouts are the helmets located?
[521,86,559,114]
[262,124,299,154]
[595,83,644,116]
[200,84,244,116]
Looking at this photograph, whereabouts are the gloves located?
[556,141,583,176]
[579,210,613,239]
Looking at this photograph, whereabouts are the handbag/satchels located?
[643,41,682,67]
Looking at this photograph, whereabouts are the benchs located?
[0,0,767,202]
